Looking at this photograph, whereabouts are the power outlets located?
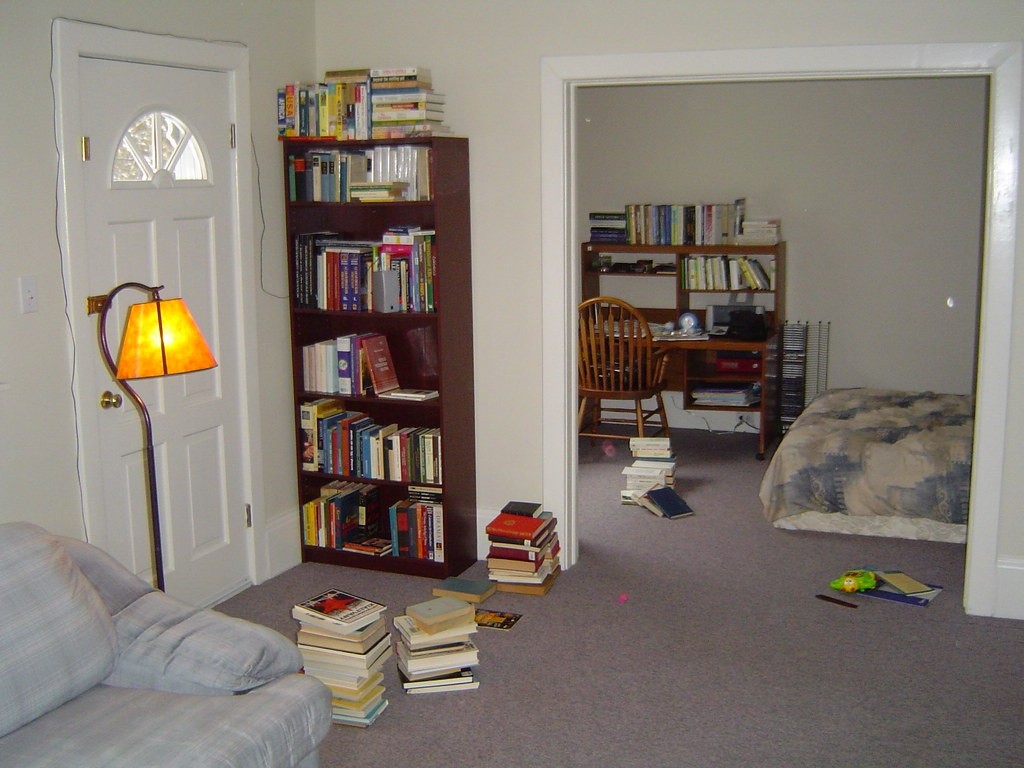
[736,412,752,425]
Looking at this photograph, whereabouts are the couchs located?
[0,524,333,768]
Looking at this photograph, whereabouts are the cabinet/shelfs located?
[580,242,788,462]
[276,135,480,585]
[778,319,832,434]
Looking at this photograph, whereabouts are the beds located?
[758,386,976,545]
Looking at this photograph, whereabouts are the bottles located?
[601,439,615,457]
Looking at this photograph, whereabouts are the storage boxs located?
[717,352,762,374]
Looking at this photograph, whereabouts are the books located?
[290,588,393,729]
[855,570,944,607]
[289,145,434,202]
[277,66,454,141]
[682,254,777,291]
[300,398,442,485]
[620,438,694,520]
[486,501,561,596]
[302,326,439,402]
[690,383,761,406]
[780,323,806,436]
[393,575,524,695]
[302,479,445,563]
[294,226,435,313]
[589,198,783,245]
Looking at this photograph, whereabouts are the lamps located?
[96,280,221,595]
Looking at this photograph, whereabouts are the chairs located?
[576,296,673,438]
